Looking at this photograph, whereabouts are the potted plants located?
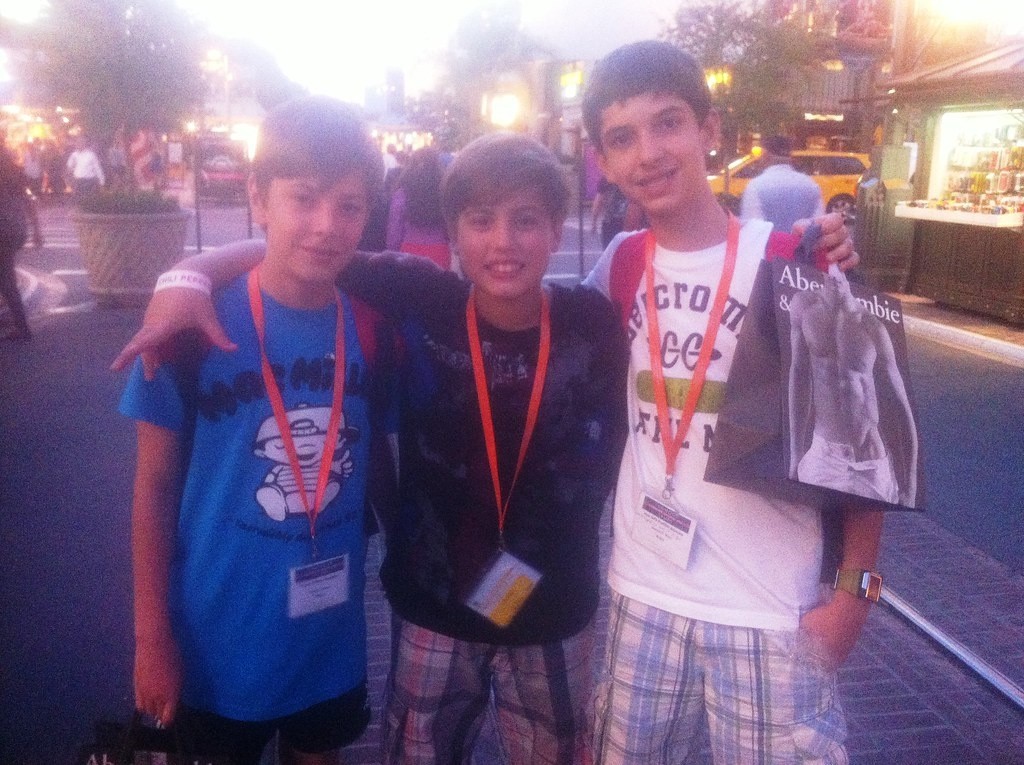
[35,0,203,305]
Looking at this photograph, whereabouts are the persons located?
[383,128,454,270]
[741,134,824,235]
[580,39,883,765]
[591,175,650,251]
[0,132,106,345]
[111,133,862,765]
[120,96,442,763]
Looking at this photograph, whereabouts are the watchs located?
[831,568,883,602]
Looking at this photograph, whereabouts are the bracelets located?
[153,270,214,295]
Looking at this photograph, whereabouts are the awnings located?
[841,41,1024,104]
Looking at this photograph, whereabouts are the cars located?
[200,144,251,208]
[709,151,871,226]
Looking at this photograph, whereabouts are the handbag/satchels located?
[704,223,927,512]
[78,708,237,765]
